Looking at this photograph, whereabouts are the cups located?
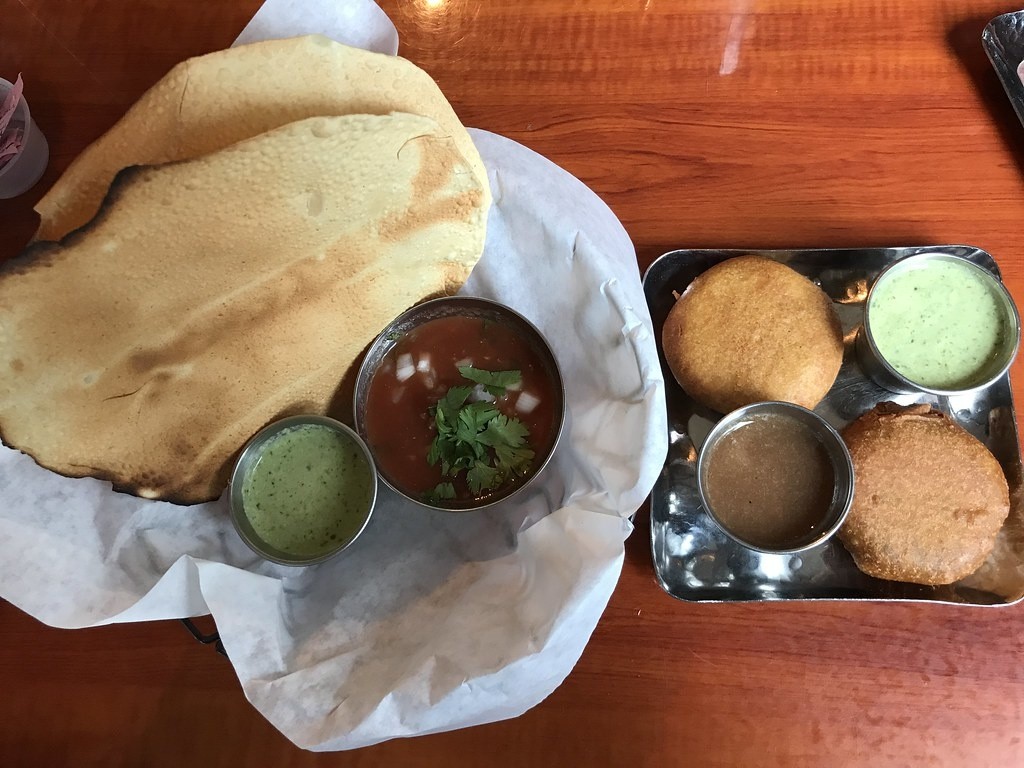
[1,78,48,204]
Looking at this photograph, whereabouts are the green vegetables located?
[423,366,535,507]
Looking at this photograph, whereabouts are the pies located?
[3,37,490,505]
[662,253,845,415]
[837,400,1011,585]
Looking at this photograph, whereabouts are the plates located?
[640,240,1023,612]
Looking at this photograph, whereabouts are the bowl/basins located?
[227,415,378,568]
[700,402,855,555]
[353,295,567,517]
[864,253,1024,398]
